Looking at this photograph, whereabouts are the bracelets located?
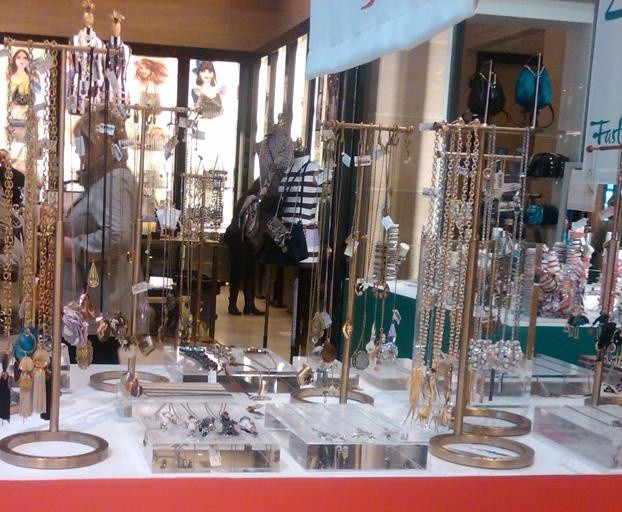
[372,221,406,285]
[598,241,622,363]
[473,226,594,317]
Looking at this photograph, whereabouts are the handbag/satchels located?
[12,82,36,105]
[274,160,309,263]
[515,66,551,109]
[526,204,544,225]
[468,73,504,115]
[238,144,288,250]
[266,160,302,247]
[529,153,568,178]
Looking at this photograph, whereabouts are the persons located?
[127,59,169,148]
[225,177,266,317]
[7,49,41,141]
[54,107,148,364]
[191,61,226,141]
[278,155,325,263]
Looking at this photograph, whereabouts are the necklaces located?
[135,112,189,358]
[138,402,258,447]
[278,398,410,443]
[206,341,263,399]
[0,141,61,419]
[243,344,295,390]
[177,343,219,373]
[180,171,225,244]
[317,120,530,429]
